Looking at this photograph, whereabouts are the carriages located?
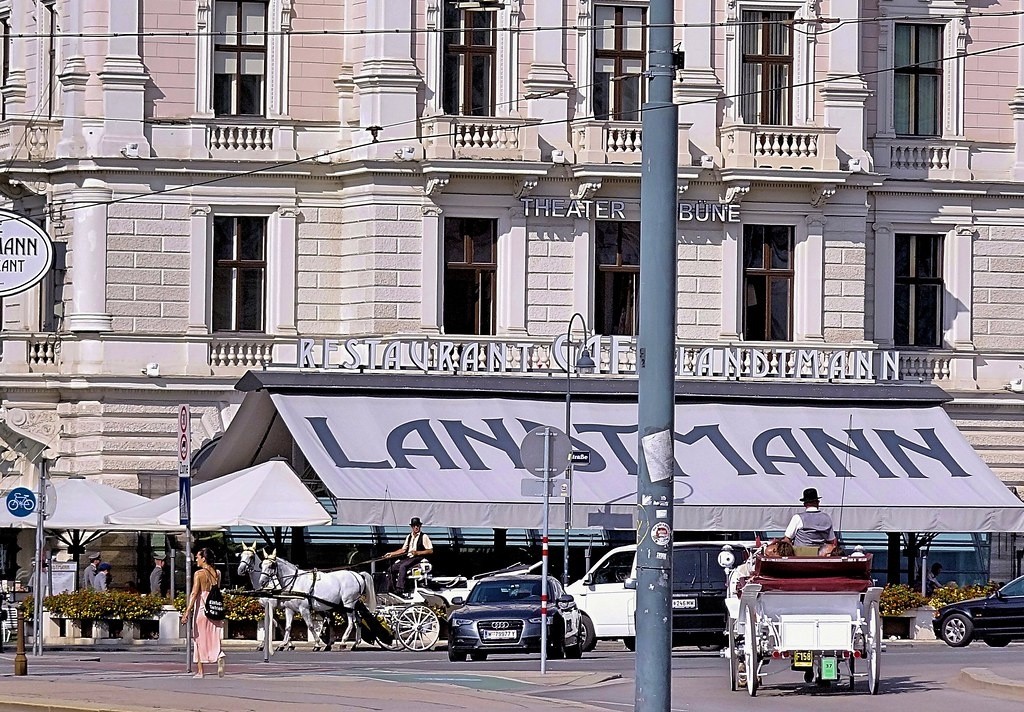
[236,540,544,658]
[716,535,885,697]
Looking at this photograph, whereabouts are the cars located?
[931,575,1024,648]
[446,574,582,663]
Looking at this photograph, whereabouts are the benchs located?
[736,552,873,597]
[383,557,432,598]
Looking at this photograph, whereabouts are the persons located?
[94,563,111,593]
[783,488,837,559]
[149,554,167,596]
[927,562,944,588]
[83,551,101,590]
[180,548,227,678]
[383,518,433,596]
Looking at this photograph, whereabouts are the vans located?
[563,540,773,652]
[623,544,749,646]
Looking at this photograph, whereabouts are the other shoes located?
[217,659,224,678]
[193,673,204,679]
[394,588,404,594]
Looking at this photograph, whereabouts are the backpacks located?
[204,567,225,621]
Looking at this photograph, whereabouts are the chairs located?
[0,607,19,643]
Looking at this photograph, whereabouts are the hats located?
[153,554,169,561]
[800,488,822,502]
[409,518,423,525]
[97,563,111,570]
[89,552,101,563]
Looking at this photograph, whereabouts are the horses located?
[237,541,334,651]
[804,544,865,685]
[729,536,782,687]
[258,547,376,652]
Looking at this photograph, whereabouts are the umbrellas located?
[0,473,153,592]
[105,454,332,552]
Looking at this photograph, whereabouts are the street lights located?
[562,312,596,584]
